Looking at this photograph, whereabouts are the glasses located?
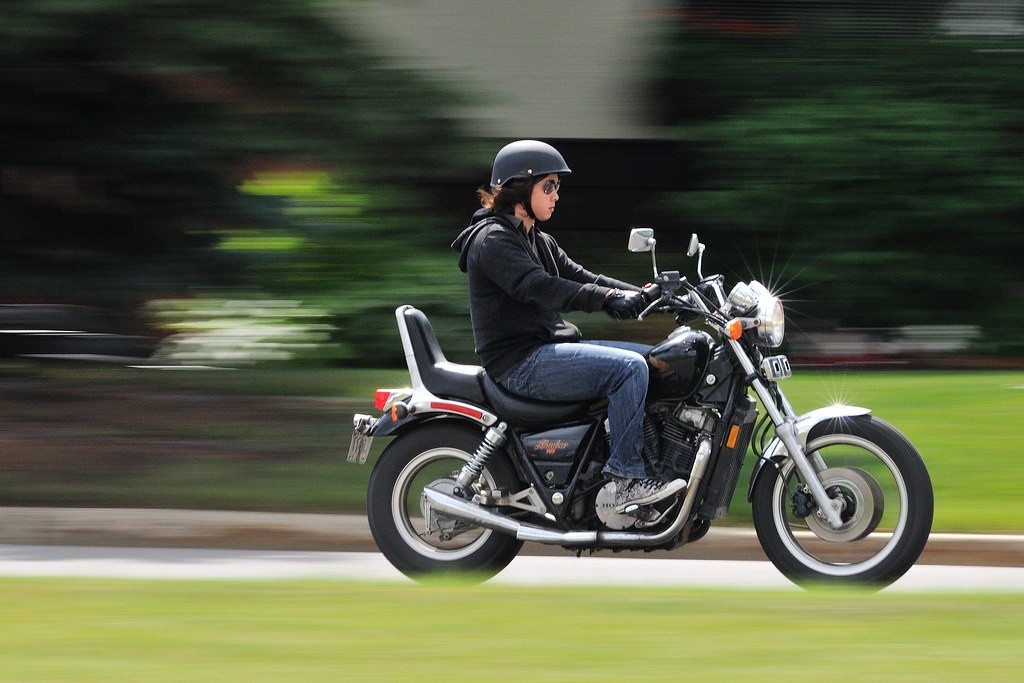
[536,179,561,194]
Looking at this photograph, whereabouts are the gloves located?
[674,286,700,323]
[601,287,651,320]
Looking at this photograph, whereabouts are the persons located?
[451,140,687,514]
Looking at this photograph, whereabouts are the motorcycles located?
[346,229,937,592]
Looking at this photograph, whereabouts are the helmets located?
[490,140,572,188]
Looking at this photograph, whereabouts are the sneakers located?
[615,476,687,514]
